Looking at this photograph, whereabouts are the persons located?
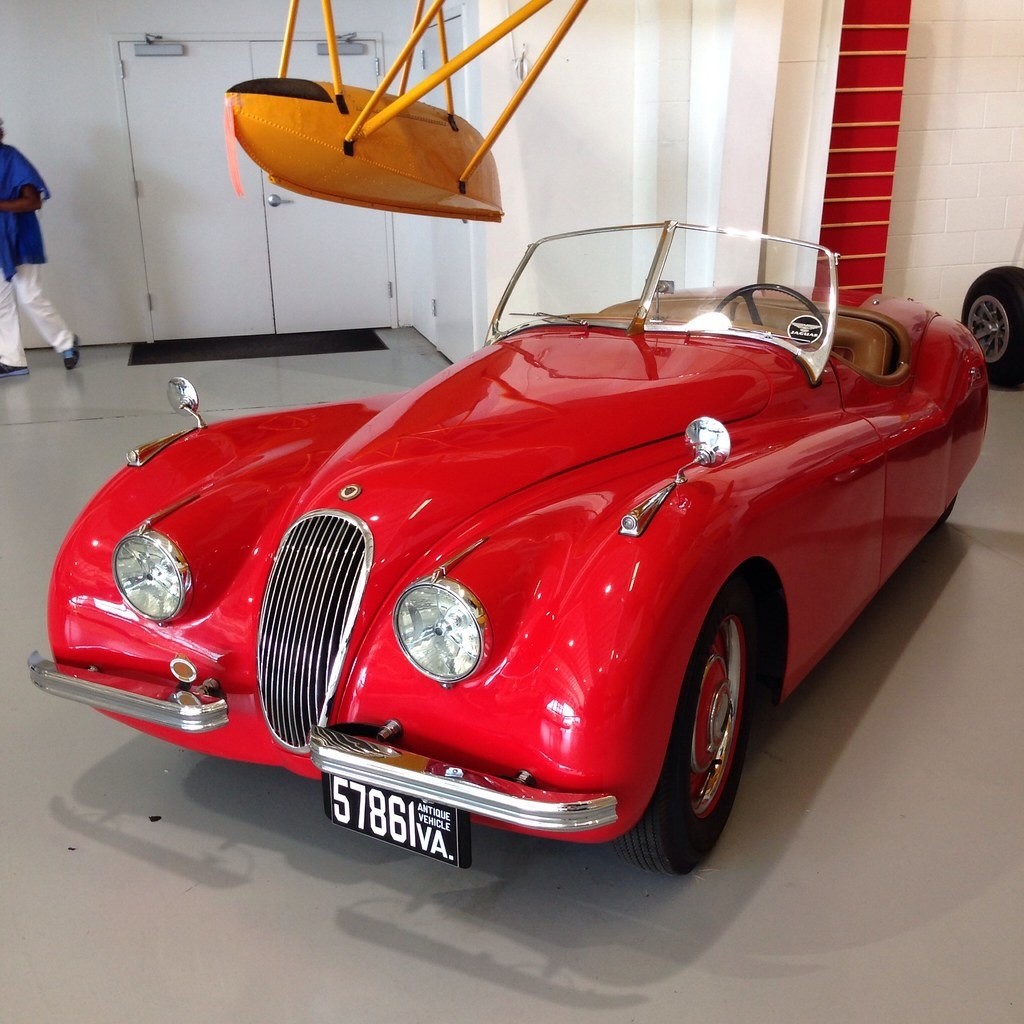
[0,118,80,376]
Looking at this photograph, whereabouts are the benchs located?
[597,295,896,386]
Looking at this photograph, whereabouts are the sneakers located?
[0,363,29,377]
[63,334,79,369]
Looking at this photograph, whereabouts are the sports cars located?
[24,218,987,879]
